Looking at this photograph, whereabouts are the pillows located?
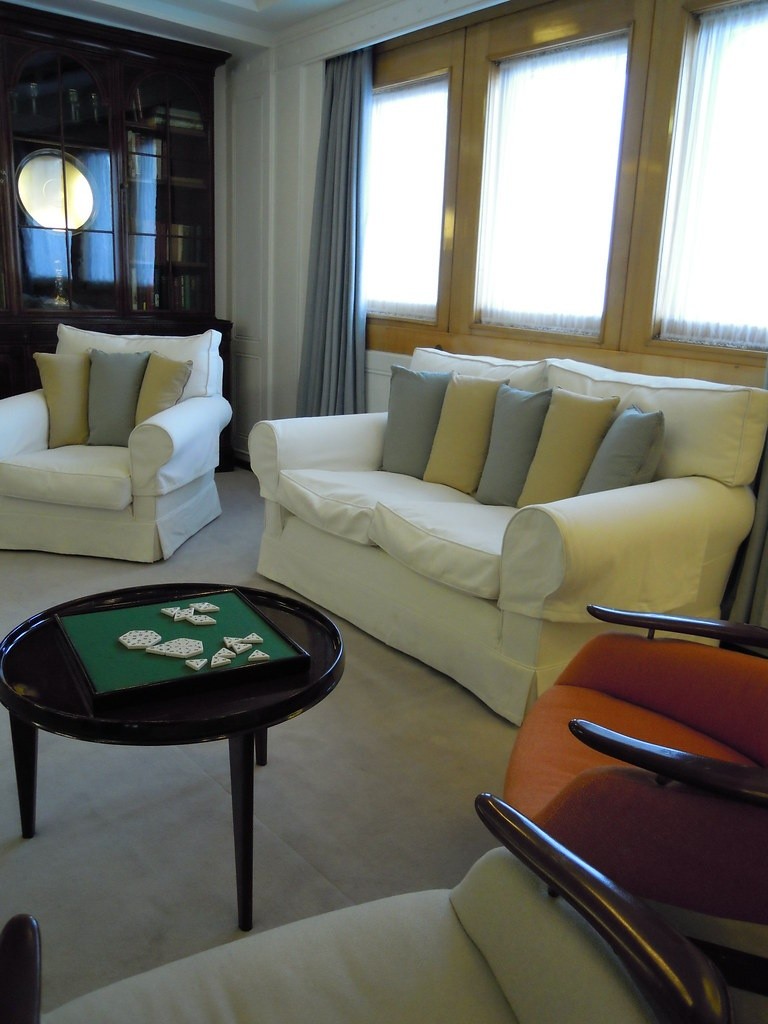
[85,348,151,449]
[474,383,556,507]
[134,348,194,427]
[422,372,511,497]
[33,351,90,450]
[579,405,665,496]
[381,364,451,482]
[517,389,621,508]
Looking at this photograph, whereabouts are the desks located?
[0,582,346,933]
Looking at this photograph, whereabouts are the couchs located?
[247,347,768,729]
[0,324,233,563]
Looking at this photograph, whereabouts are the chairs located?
[0,792,718,1024]
[504,606,768,991]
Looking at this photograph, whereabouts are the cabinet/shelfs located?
[0,0,238,471]
